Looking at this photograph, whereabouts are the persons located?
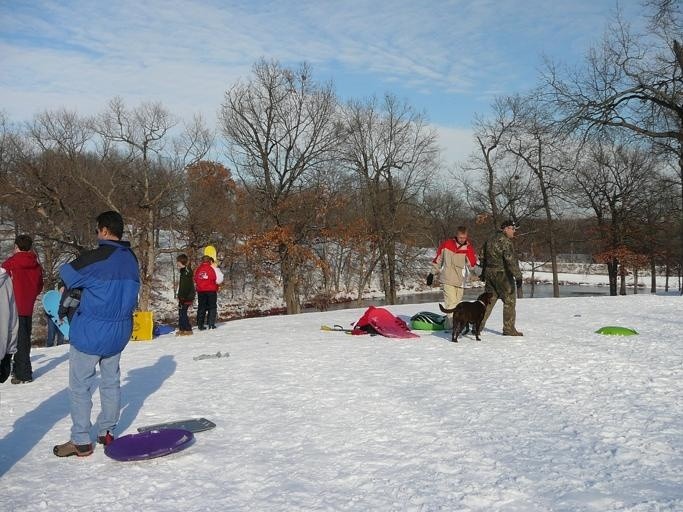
[176,254,195,335]
[44,285,65,347]
[53,211,141,457]
[427,226,478,334]
[472,220,523,336]
[2,234,43,384]
[0,267,19,378]
[195,255,216,330]
[196,258,214,326]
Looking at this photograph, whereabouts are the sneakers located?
[177,331,192,335]
[198,325,206,330]
[11,379,31,384]
[53,441,92,457]
[97,431,114,445]
[504,330,522,336]
[209,326,216,328]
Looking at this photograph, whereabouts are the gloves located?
[427,274,433,285]
[516,279,522,288]
[478,275,485,281]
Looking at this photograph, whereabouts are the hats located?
[501,221,519,229]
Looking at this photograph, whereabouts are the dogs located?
[439,292,494,342]
